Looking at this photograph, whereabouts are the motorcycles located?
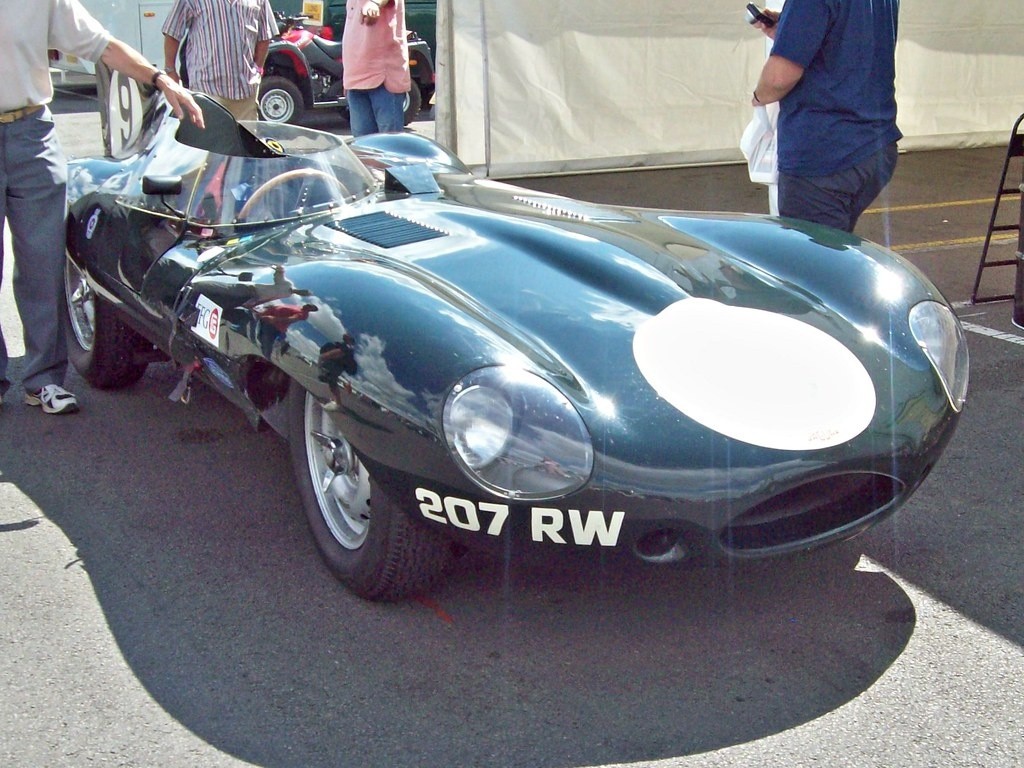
[256,8,438,126]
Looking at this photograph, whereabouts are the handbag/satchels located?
[738,101,780,185]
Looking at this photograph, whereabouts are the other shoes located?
[319,400,344,413]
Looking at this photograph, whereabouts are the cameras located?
[745,2,775,29]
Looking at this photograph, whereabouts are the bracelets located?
[754,91,760,103]
[152,70,166,90]
[257,68,264,76]
[164,65,176,75]
[370,0,381,8]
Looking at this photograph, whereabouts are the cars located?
[64,86,972,605]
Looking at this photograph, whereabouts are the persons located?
[752,0,904,233]
[162,0,280,137]
[342,0,411,137]
[0,0,204,413]
[184,265,358,412]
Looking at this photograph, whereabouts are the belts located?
[0,105,41,125]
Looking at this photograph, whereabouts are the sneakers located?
[25,383,80,413]
